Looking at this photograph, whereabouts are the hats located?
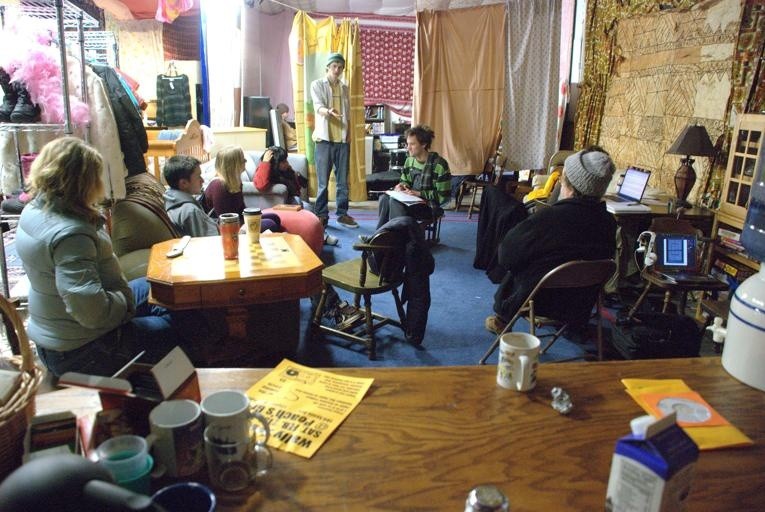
[564,146,615,197]
[327,53,345,66]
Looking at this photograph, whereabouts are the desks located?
[148,232,323,366]
[473,168,707,315]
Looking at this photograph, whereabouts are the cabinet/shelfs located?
[364,104,390,136]
[0,0,119,358]
[694,114,765,331]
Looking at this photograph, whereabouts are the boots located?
[10,76,42,123]
[0,71,17,119]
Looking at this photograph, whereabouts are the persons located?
[310,53,359,228]
[198,146,280,233]
[358,127,453,244]
[15,137,219,380]
[161,156,220,237]
[253,146,338,245]
[485,145,617,336]
[277,103,296,148]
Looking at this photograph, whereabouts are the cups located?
[496,333,541,392]
[219,212,239,259]
[242,208,263,243]
[93,387,271,511]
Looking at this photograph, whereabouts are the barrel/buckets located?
[738,129,765,263]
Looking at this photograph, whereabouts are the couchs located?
[111,170,324,283]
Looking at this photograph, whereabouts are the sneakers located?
[336,214,359,228]
[486,314,512,337]
[323,233,338,245]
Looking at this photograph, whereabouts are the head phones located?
[636,231,657,266]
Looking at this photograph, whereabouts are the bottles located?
[464,486,509,512]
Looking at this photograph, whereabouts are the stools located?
[416,209,441,248]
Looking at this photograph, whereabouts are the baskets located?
[0,296,45,455]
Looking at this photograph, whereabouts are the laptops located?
[600,166,651,205]
[655,234,718,283]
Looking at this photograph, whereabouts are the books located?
[368,190,427,208]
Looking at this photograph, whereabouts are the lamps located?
[665,126,717,209]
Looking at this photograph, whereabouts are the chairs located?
[455,153,508,219]
[622,218,731,323]
[198,150,309,210]
[478,262,617,362]
[308,233,411,357]
